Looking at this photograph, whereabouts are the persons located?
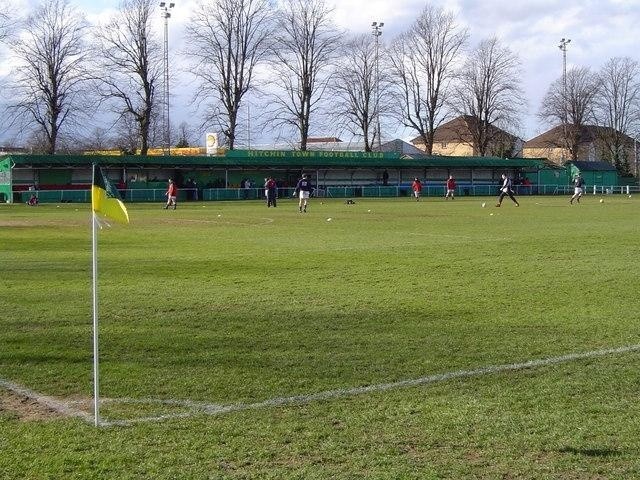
[383,170,389,185]
[293,173,311,213]
[445,173,456,200]
[117,177,126,200]
[567,173,586,205]
[514,174,531,187]
[162,176,282,210]
[28,196,39,206]
[494,171,520,207]
[412,178,422,201]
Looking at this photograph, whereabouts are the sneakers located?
[164,205,176,210]
[570,199,579,204]
[299,209,306,212]
[496,204,500,207]
[516,204,519,207]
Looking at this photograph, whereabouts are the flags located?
[91,164,131,224]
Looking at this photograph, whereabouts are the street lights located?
[372,21,384,150]
[159,2,174,156]
[559,39,571,165]
